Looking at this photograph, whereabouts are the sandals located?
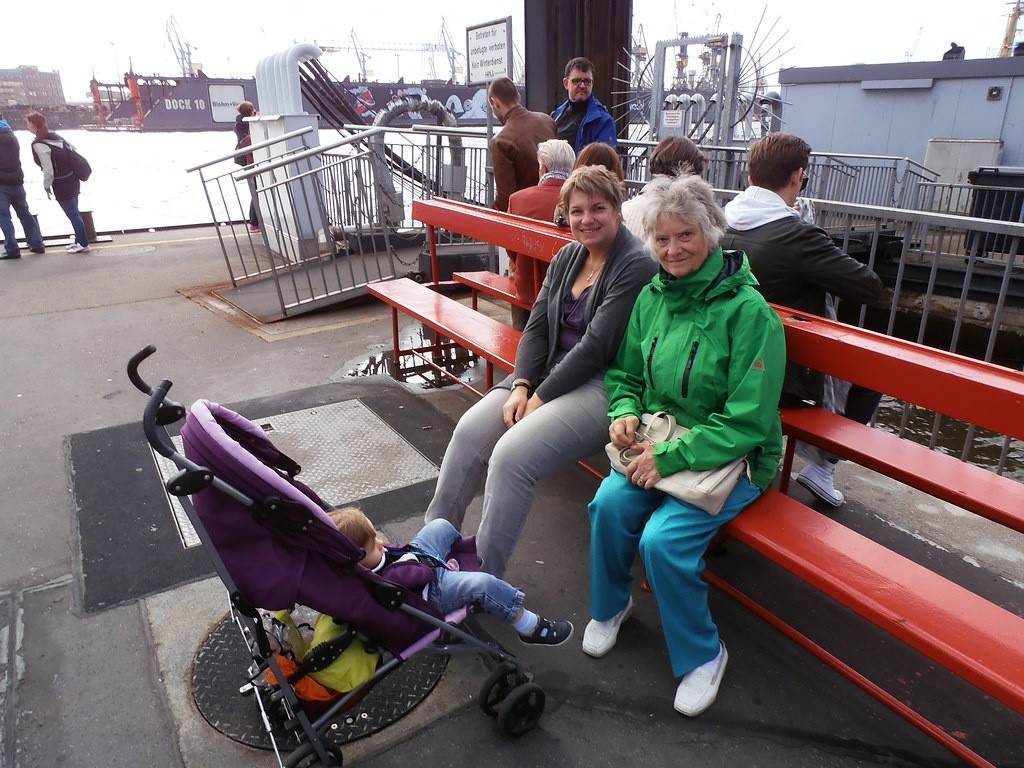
[518,614,574,647]
[475,556,484,568]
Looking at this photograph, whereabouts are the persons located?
[581,160,786,718]
[505,139,576,333]
[0,112,45,260]
[232,101,261,233]
[26,111,90,255]
[720,132,883,508]
[423,165,661,581]
[324,506,574,647]
[486,77,558,213]
[547,56,617,155]
[574,141,624,183]
[619,136,704,256]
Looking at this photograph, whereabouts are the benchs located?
[365,197,1024,768]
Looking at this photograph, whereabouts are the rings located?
[637,479,644,486]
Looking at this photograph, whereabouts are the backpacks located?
[36,131,92,181]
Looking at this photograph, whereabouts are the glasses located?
[793,167,809,191]
[567,78,593,86]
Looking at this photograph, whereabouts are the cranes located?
[699,11,721,87]
[294,39,449,80]
[166,15,203,77]
[350,29,371,78]
[440,20,458,83]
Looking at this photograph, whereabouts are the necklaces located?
[587,256,604,282]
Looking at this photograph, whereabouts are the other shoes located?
[0,252,20,259]
[30,246,45,253]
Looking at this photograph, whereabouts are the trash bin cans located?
[962,166,1024,264]
[819,223,905,331]
[701,170,809,208]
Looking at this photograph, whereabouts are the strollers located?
[127,345,546,768]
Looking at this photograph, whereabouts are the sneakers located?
[583,596,633,657]
[67,243,90,253]
[674,637,728,717]
[249,223,262,233]
[796,456,844,507]
[65,242,77,249]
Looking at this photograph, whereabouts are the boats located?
[80,73,748,131]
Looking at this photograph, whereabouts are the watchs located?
[511,381,530,396]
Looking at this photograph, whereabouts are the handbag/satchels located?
[605,411,752,516]
[234,154,247,166]
[274,608,379,694]
